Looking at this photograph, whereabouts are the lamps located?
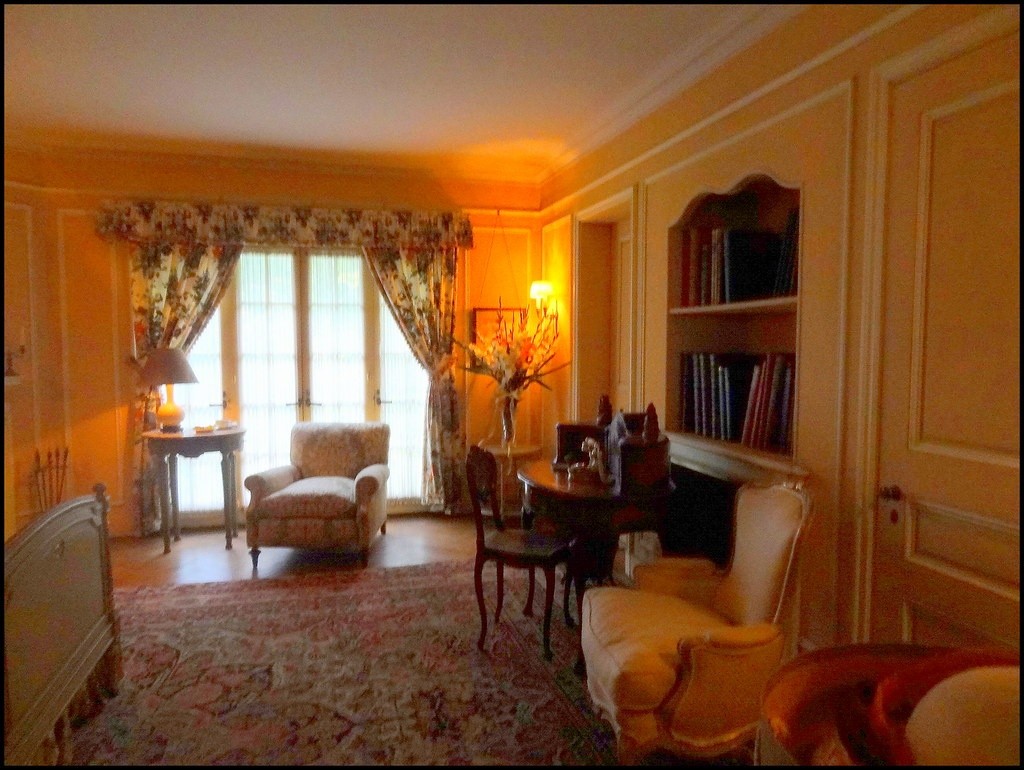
[141,348,199,434]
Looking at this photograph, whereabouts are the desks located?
[516,457,654,617]
[483,443,543,520]
[142,430,247,554]
[753,641,932,767]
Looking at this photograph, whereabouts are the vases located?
[500,395,516,452]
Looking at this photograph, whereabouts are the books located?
[679,223,796,457]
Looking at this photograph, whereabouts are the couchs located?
[581,480,815,767]
[243,421,391,569]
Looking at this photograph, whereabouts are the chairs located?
[466,444,582,660]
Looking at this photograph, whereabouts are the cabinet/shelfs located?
[667,295,798,484]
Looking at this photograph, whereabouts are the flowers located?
[453,298,571,399]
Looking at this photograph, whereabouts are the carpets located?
[31,555,617,767]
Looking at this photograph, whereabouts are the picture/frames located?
[471,307,527,370]
